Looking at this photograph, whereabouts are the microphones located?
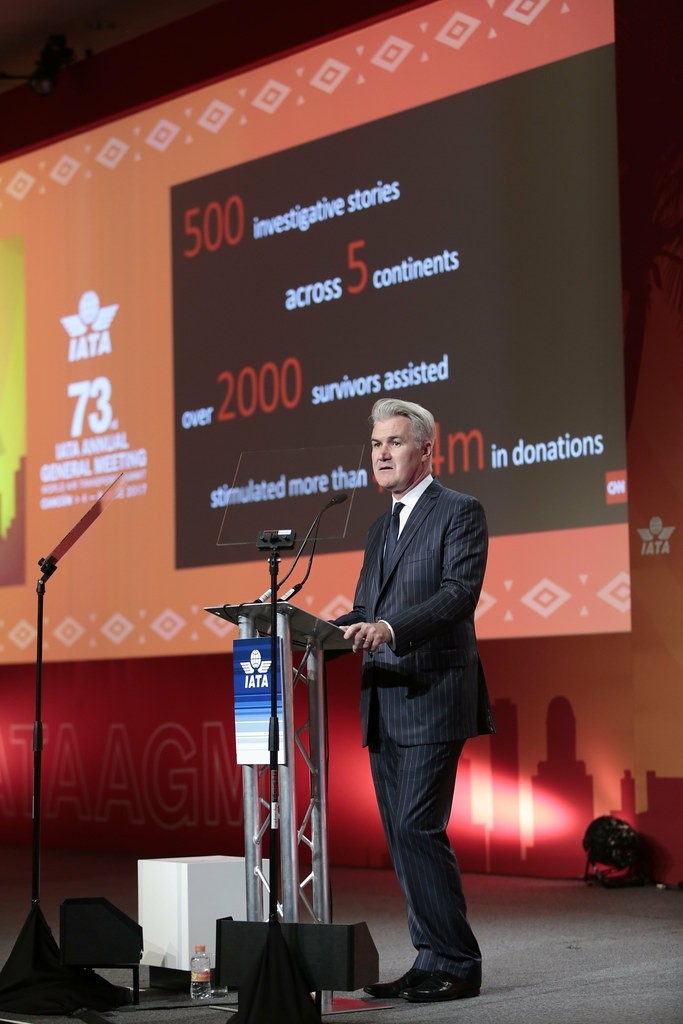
[252,493,349,605]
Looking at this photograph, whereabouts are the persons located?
[325,398,494,1005]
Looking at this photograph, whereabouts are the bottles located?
[190,944,211,999]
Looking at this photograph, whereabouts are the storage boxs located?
[137,851,271,973]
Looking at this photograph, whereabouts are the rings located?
[366,638,371,643]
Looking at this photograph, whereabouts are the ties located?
[382,502,406,585]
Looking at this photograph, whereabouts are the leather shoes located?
[363,968,433,998]
[399,973,480,1002]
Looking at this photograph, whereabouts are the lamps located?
[582,815,652,889]
